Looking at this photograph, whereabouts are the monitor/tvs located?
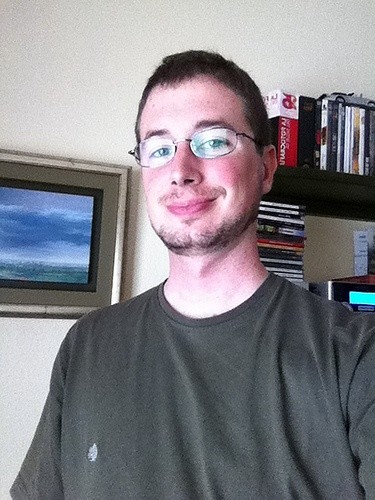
[328,281,375,313]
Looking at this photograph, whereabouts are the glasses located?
[128,127,256,168]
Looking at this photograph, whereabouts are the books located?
[262,91,375,176]
[258,201,308,291]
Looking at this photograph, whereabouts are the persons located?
[10,49,375,500]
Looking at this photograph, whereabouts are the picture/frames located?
[0,147,132,320]
